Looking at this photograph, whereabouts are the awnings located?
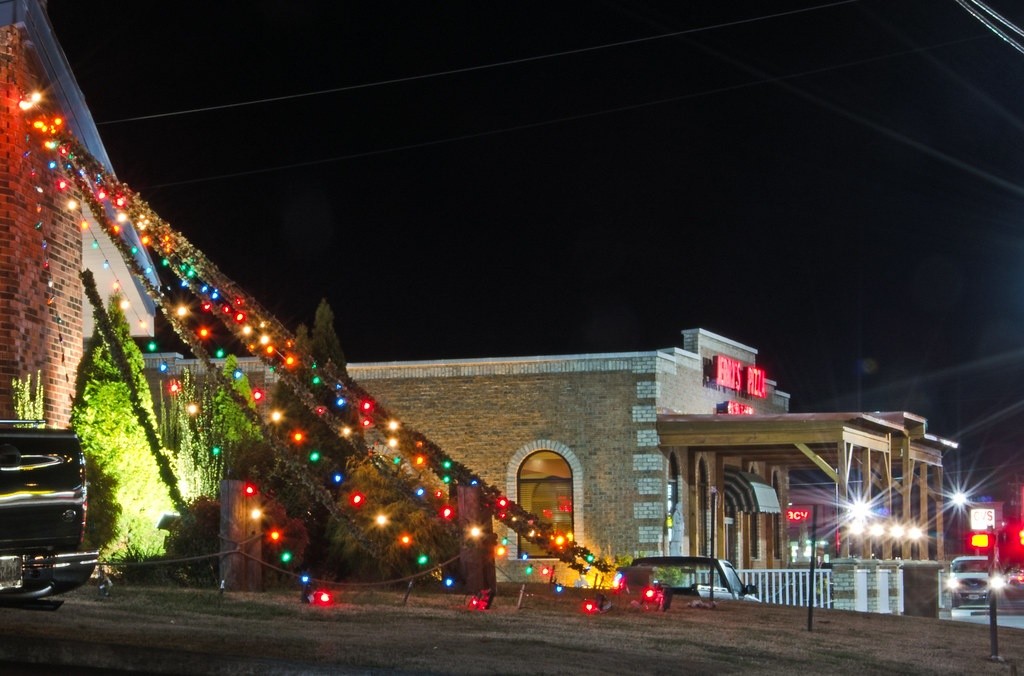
[724,468,781,514]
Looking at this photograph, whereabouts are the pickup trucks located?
[618,556,760,602]
[0,418,101,617]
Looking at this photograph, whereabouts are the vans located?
[947,556,1001,607]
